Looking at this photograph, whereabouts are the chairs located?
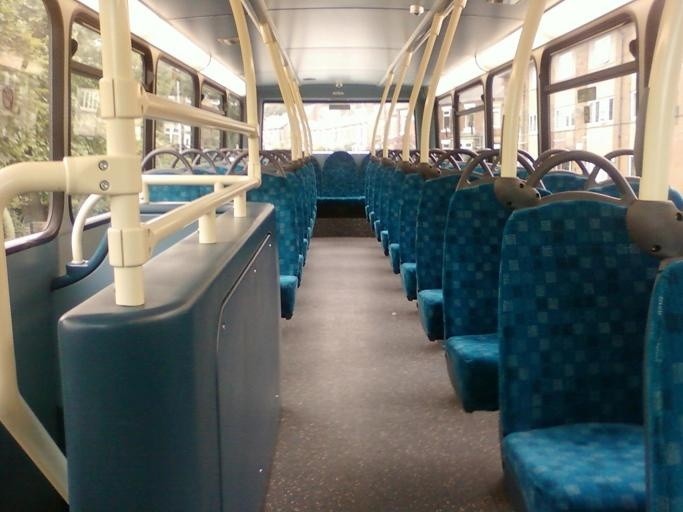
[322,151,361,200]
[362,147,681,511]
[137,148,320,319]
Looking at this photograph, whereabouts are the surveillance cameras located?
[409,5,425,17]
